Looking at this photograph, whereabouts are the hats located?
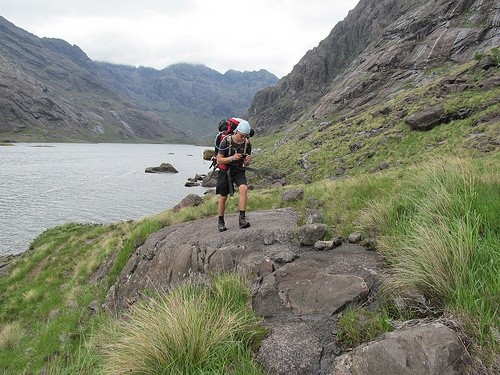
[237,121,250,134]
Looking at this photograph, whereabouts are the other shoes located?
[217,220,227,232]
[239,215,250,229]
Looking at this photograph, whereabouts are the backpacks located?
[208,118,254,181]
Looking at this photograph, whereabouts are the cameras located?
[241,155,247,158]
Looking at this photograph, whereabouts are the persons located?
[216,121,252,232]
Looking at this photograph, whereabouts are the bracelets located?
[230,156,234,161]
